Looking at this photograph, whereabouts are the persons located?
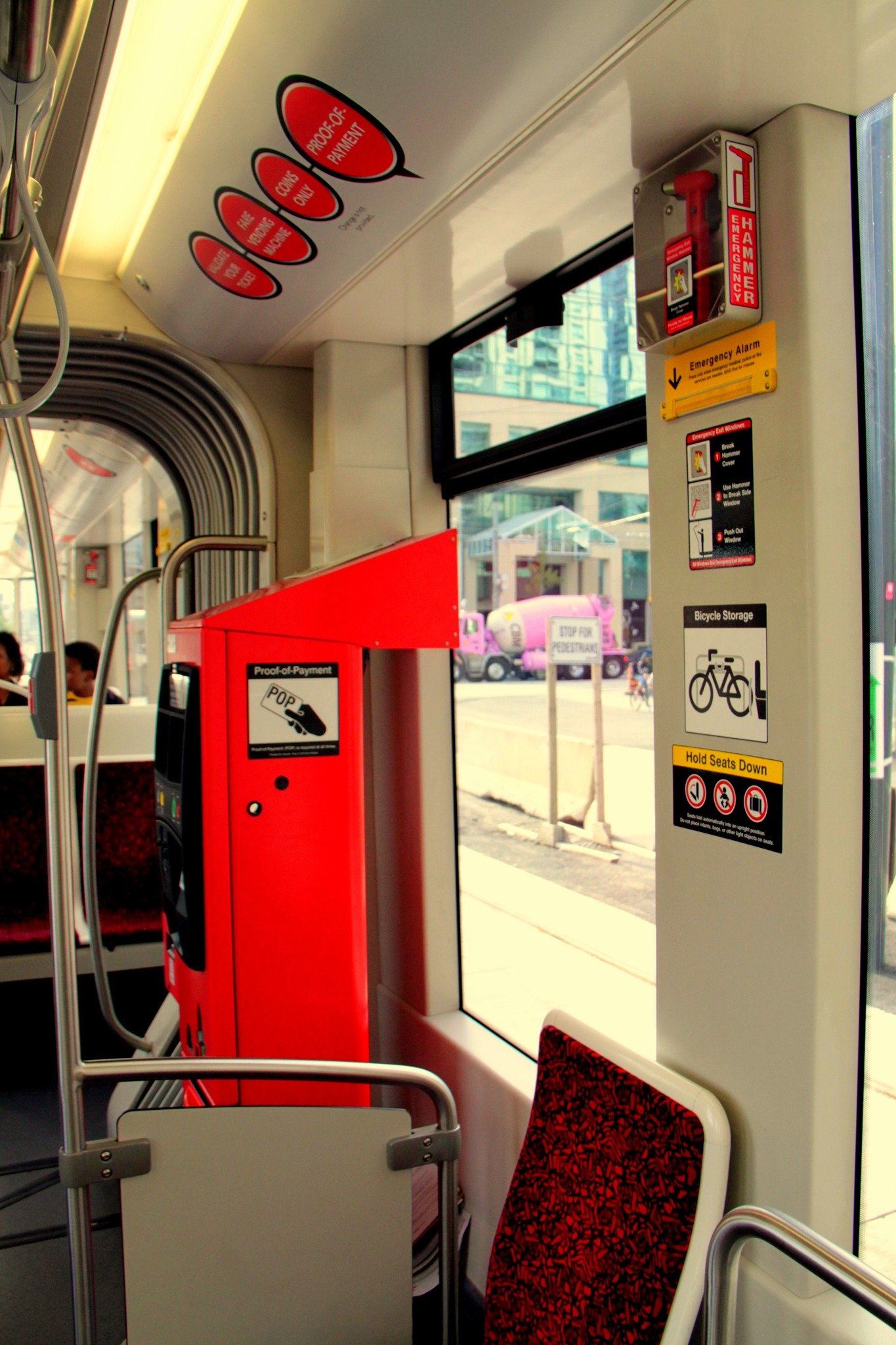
[0,632,123,706]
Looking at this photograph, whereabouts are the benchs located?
[0,758,167,983]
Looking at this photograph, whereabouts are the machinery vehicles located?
[454,594,634,682]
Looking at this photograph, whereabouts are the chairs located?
[485,1008,732,1345]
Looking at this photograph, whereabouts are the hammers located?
[662,170,716,325]
